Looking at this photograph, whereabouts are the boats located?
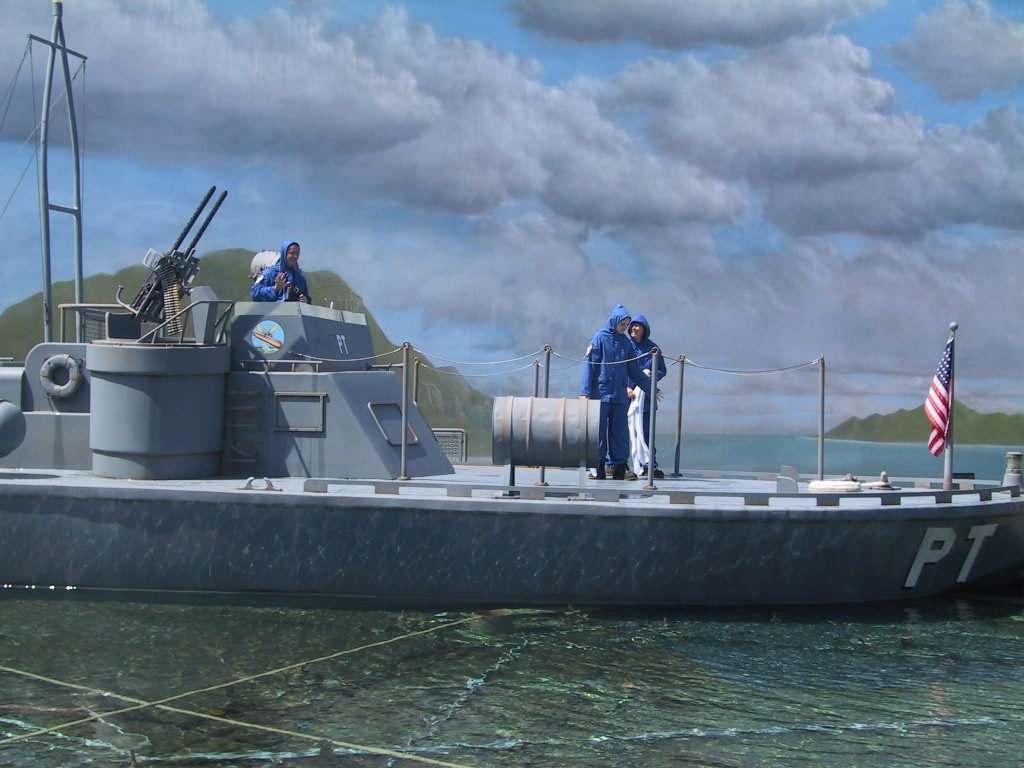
[0,0,1023,620]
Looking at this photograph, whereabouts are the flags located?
[923,322,958,456]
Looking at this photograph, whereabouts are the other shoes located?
[623,468,638,480]
[588,471,606,480]
[644,467,663,478]
[612,465,625,480]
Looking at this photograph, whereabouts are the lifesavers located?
[41,354,81,400]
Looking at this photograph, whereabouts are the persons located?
[625,314,666,480]
[250,240,312,305]
[579,304,666,481]
